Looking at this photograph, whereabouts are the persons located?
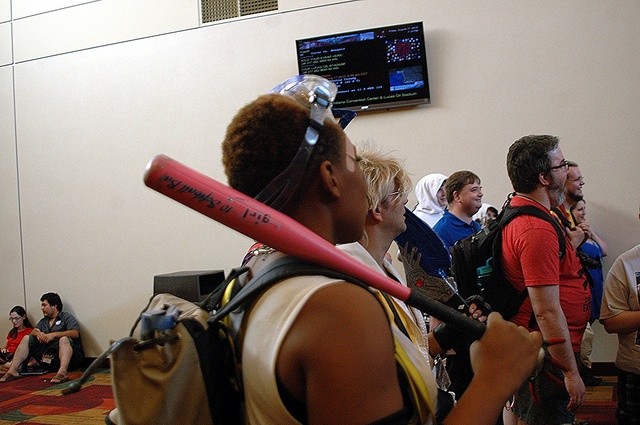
[206,94,542,425]
[502,135,592,425]
[429,170,483,403]
[549,160,594,289]
[0,293,85,384]
[0,306,34,377]
[397,173,449,263]
[569,198,609,386]
[1,299,40,378]
[335,141,491,425]
[472,203,498,230]
[599,204,640,425]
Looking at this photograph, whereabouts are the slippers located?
[50,373,69,384]
[0,371,22,383]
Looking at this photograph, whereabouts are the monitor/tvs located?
[295,20,431,115]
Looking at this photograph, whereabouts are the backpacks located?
[450,199,566,329]
[549,207,594,290]
[60,255,457,425]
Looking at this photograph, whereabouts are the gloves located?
[431,295,492,355]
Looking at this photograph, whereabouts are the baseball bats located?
[143,154,545,368]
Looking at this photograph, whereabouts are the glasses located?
[8,315,25,320]
[238,75,337,204]
[550,160,571,170]
[381,191,401,204]
